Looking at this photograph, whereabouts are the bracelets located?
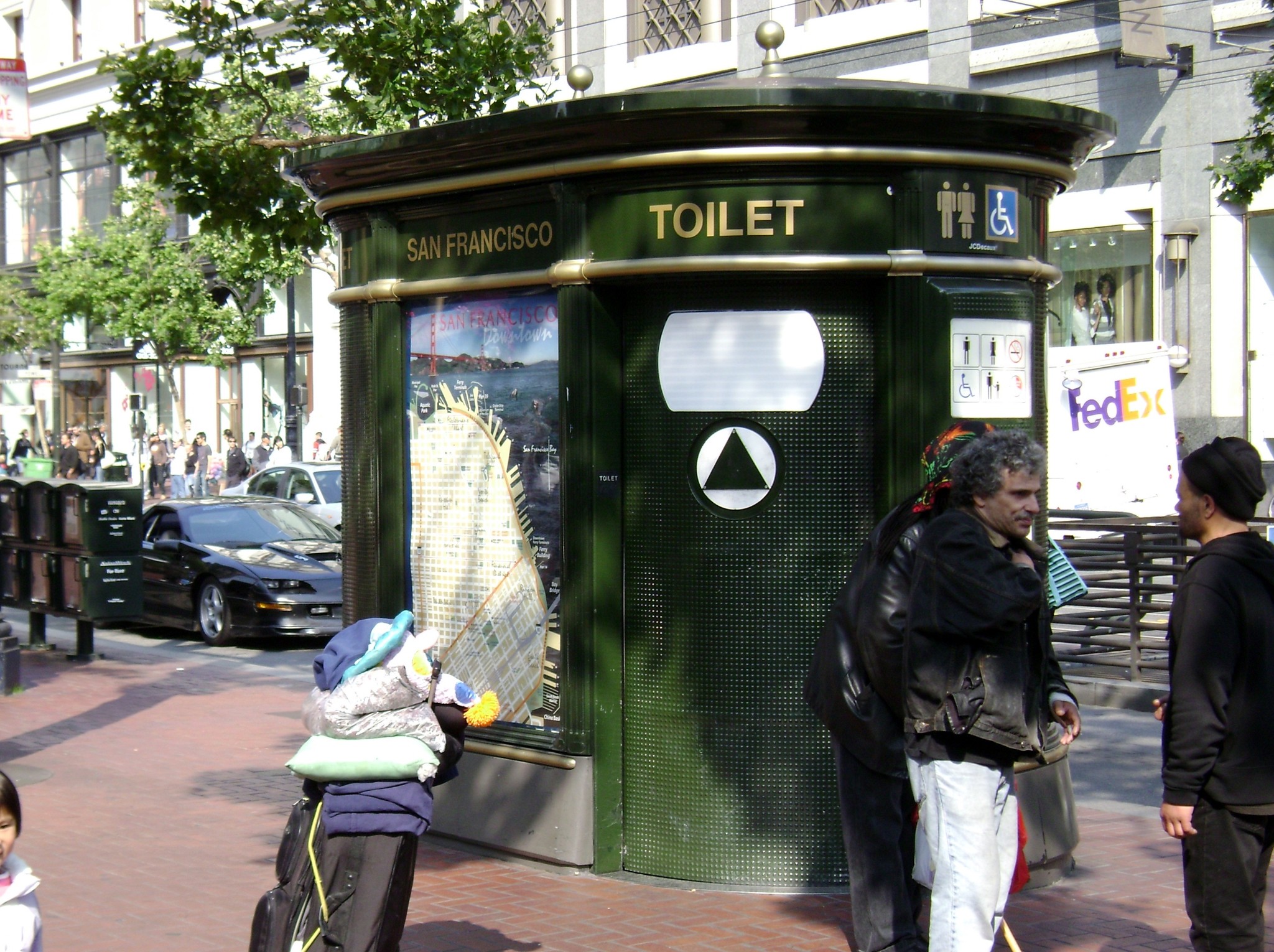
[206,473,210,474]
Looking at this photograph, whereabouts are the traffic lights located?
[129,395,147,410]
[290,386,307,407]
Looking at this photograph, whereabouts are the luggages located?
[247,659,442,952]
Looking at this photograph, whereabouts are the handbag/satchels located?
[98,442,116,469]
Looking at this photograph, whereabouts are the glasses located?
[229,441,236,443]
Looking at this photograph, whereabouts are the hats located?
[1181,436,1266,520]
[261,434,272,439]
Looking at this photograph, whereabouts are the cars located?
[221,460,344,536]
[124,495,344,646]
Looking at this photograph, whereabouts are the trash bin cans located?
[17,458,58,478]
[104,452,130,482]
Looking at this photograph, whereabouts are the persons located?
[1089,274,1117,344]
[0,770,42,952]
[127,419,212,500]
[903,430,1081,952]
[1073,281,1093,346]
[327,426,341,461]
[313,432,331,461]
[0,423,107,482]
[269,435,291,466]
[804,420,998,952]
[1176,432,1189,460]
[1153,435,1274,952]
[217,429,273,496]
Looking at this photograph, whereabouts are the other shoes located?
[161,495,166,500]
[144,494,154,500]
